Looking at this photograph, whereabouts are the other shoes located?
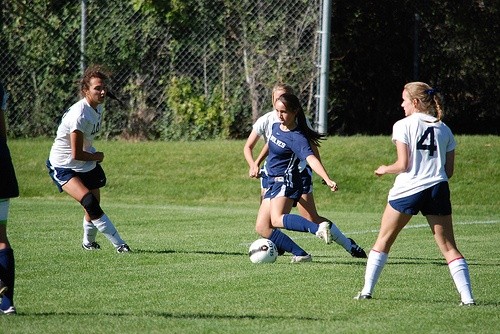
[459,302,475,306]
[0,279,8,295]
[82,241,102,251]
[354,290,372,301]
[0,306,17,315]
[116,243,132,254]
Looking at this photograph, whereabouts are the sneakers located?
[315,221,331,244]
[346,237,367,258]
[291,253,312,263]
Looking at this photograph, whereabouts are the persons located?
[0,85,20,315]
[243,83,367,258]
[353,81,475,306]
[46,72,134,253]
[249,92,339,262]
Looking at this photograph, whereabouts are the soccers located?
[248,238,279,264]
